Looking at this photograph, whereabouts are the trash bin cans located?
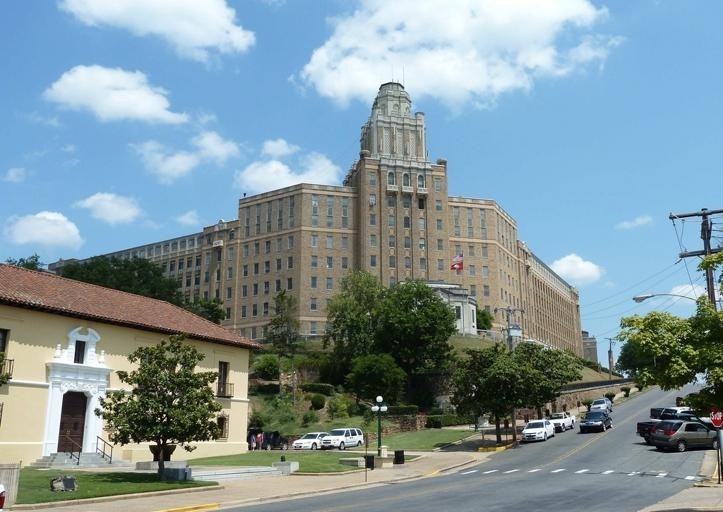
[364,456,374,470]
[395,450,404,464]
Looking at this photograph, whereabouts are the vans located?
[320,428,364,450]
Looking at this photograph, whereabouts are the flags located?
[449,252,463,271]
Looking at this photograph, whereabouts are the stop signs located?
[710,407,722,427]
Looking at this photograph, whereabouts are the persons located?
[256,431,263,451]
[250,431,256,451]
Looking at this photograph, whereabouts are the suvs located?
[248,429,288,450]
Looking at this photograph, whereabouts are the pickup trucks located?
[550,412,575,431]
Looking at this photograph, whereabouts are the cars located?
[292,432,328,449]
[590,398,612,413]
[580,412,612,431]
[522,420,555,442]
[636,406,718,452]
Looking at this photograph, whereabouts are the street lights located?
[634,294,715,313]
[371,396,388,456]
